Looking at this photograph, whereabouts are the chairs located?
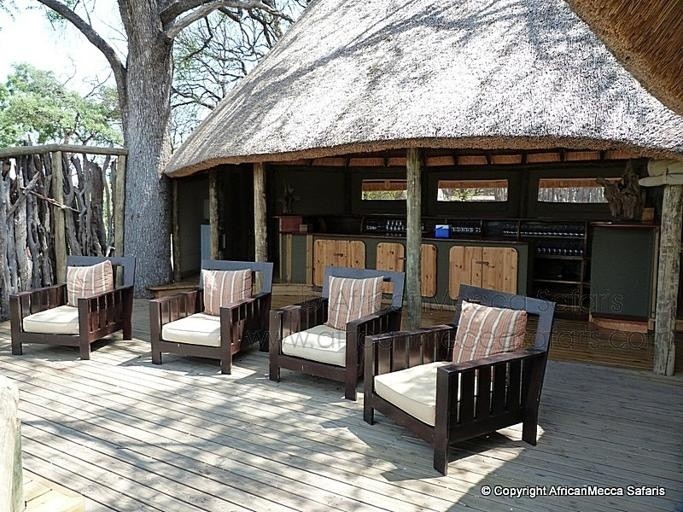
[8,256,559,476]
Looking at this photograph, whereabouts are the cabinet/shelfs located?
[360,213,594,321]
